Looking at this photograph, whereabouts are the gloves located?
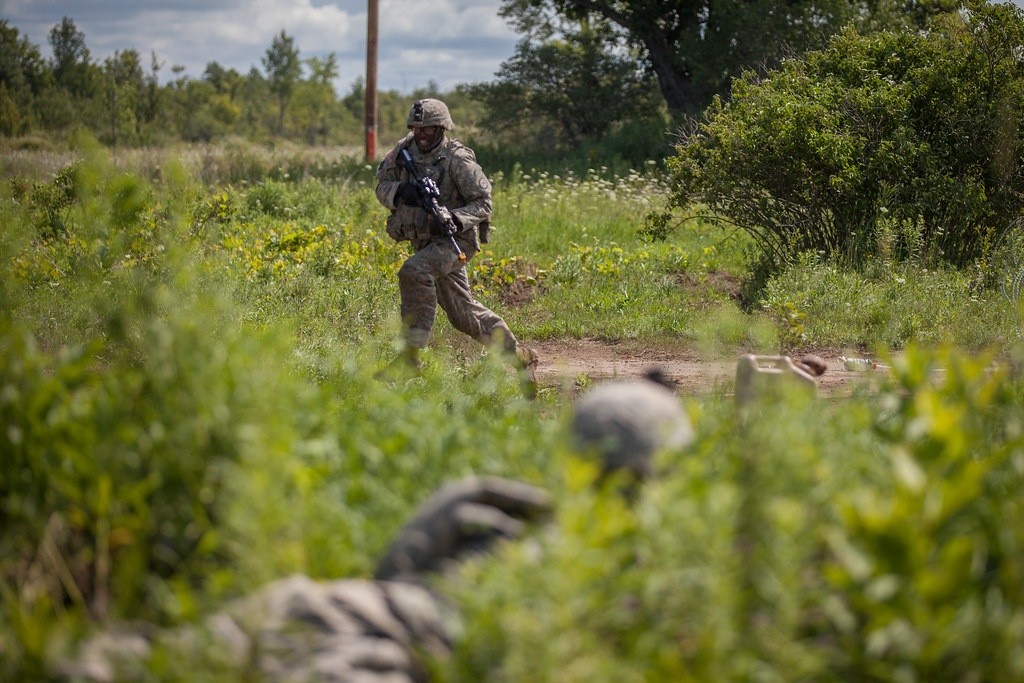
[429,215,454,236]
[397,183,424,207]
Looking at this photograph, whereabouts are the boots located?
[505,348,539,402]
[372,344,422,382]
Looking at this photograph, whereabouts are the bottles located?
[843,358,877,372]
[734,352,827,415]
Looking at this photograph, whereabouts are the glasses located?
[411,127,438,136]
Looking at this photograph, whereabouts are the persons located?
[374,99,540,401]
[73,375,694,683]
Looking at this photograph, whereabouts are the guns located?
[394,146,467,263]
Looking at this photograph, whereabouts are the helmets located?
[560,375,697,481]
[407,98,454,131]
